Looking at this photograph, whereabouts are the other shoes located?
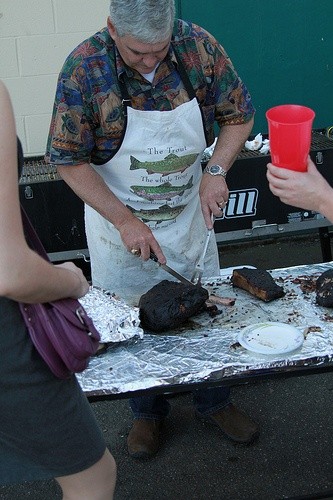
[209,402,259,444]
[126,414,164,459]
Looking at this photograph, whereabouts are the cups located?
[266,104,315,172]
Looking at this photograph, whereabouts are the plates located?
[238,322,305,354]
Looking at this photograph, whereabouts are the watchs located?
[203,164,227,178]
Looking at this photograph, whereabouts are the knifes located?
[150,252,195,285]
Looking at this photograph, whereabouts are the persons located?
[0,81,117,500]
[266,155,333,225]
[43,0,259,460]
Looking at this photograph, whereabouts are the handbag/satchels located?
[17,292,101,380]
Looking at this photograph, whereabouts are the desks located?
[72,261,333,403]
[45,216,333,280]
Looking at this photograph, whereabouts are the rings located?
[131,249,138,254]
[219,201,224,205]
